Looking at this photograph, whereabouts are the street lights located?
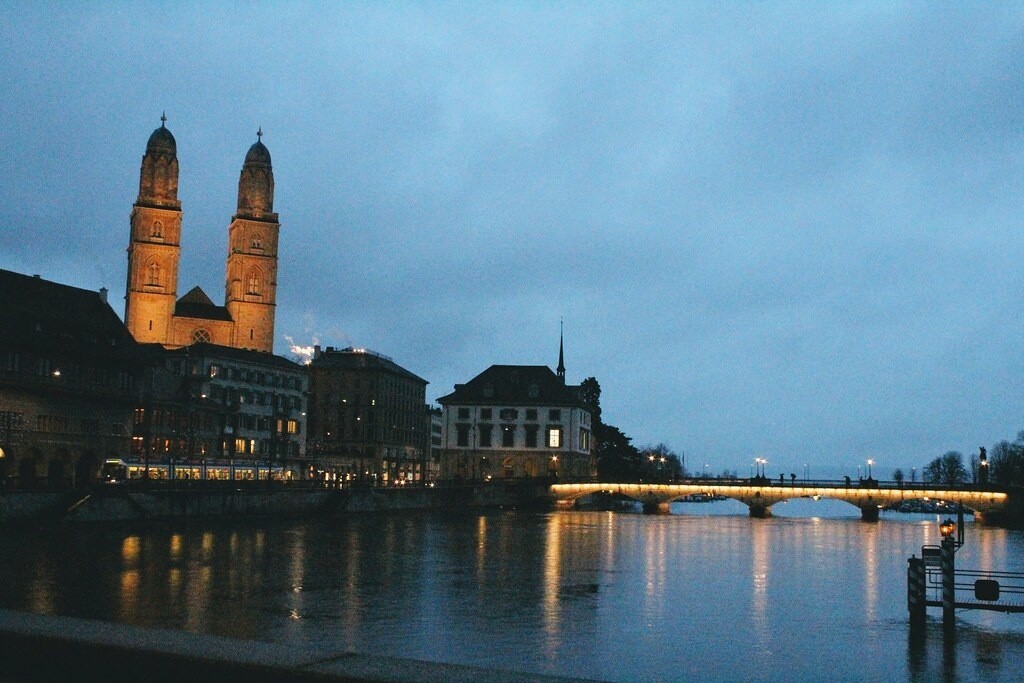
[756,458,760,484]
[761,459,766,484]
[649,456,655,482]
[867,460,872,487]
[552,455,557,481]
[660,457,665,482]
